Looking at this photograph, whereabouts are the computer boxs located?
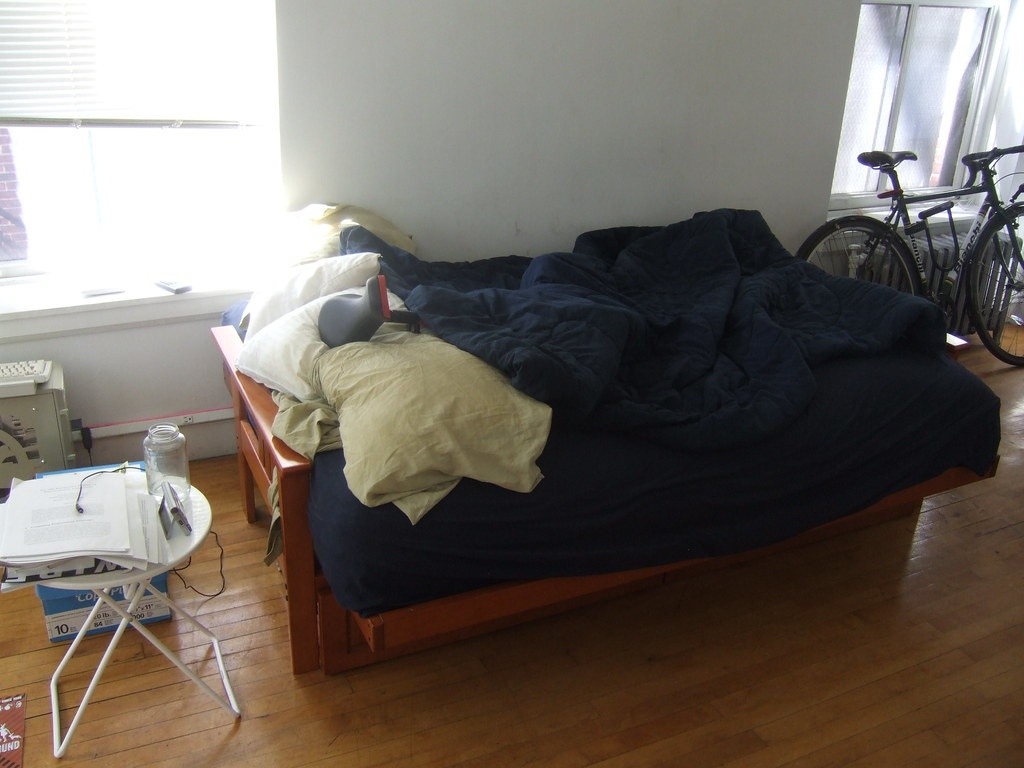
[0,368,78,488]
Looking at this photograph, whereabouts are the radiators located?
[854,232,1022,336]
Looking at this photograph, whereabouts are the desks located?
[0,475,242,759]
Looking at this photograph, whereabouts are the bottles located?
[143,422,190,516]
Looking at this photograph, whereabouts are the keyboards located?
[0,360,53,398]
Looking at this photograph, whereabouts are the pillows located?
[240,251,364,344]
[236,283,410,402]
[258,205,419,274]
[314,327,552,526]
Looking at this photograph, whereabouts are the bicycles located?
[794,145,1024,366]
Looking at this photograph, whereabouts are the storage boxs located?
[35,460,172,644]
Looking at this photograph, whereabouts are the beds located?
[211,200,1003,677]
